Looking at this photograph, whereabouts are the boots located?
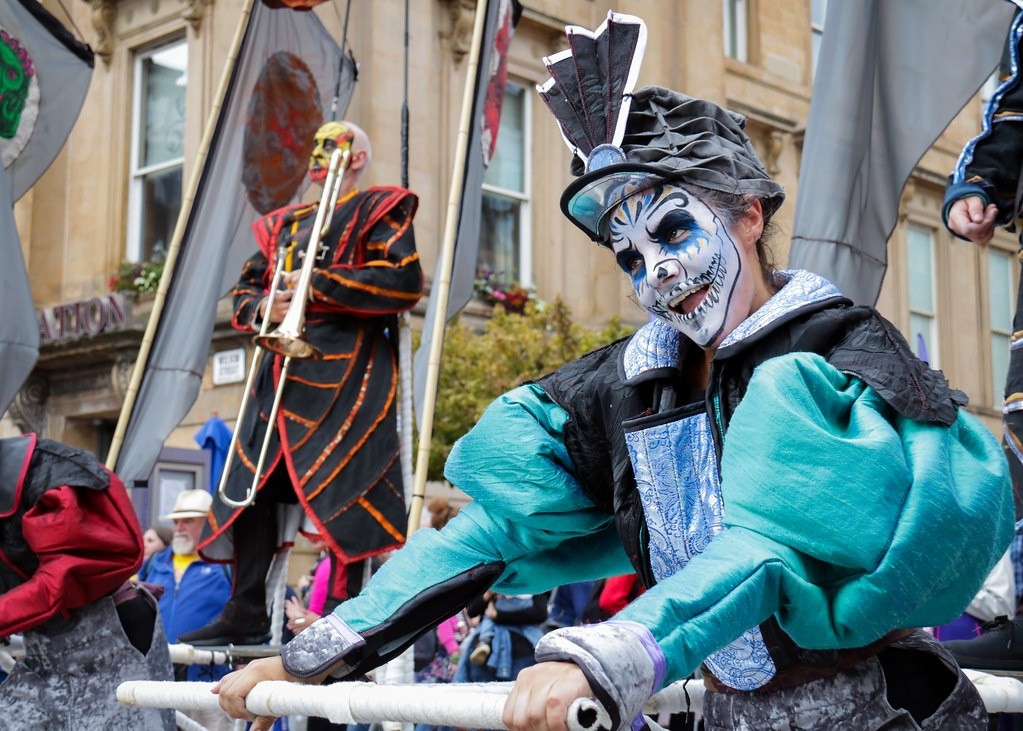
[178,495,278,645]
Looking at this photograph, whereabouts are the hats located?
[155,527,172,547]
[159,489,214,519]
[536,8,785,253]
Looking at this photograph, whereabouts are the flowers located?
[468,269,530,309]
[103,259,165,294]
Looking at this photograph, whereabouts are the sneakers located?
[939,614,1023,677]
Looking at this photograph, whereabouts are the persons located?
[941,4,1023,526]
[180,119,425,647]
[0,434,159,731]
[211,85,1012,731]
[128,489,649,731]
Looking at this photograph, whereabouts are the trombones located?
[217,149,350,510]
